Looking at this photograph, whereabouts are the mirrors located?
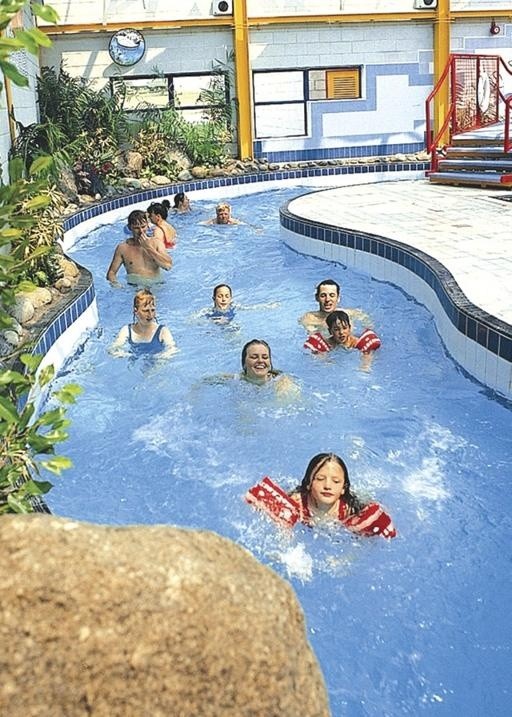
[108,28,146,67]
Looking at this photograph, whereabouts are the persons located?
[147,203,176,249]
[109,290,175,363]
[191,285,281,336]
[301,280,370,337]
[108,210,172,290]
[276,453,358,527]
[316,310,373,373]
[172,193,212,222]
[200,204,247,227]
[196,339,302,406]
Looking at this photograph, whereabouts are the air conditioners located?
[413,0,438,9]
[212,0,233,16]
